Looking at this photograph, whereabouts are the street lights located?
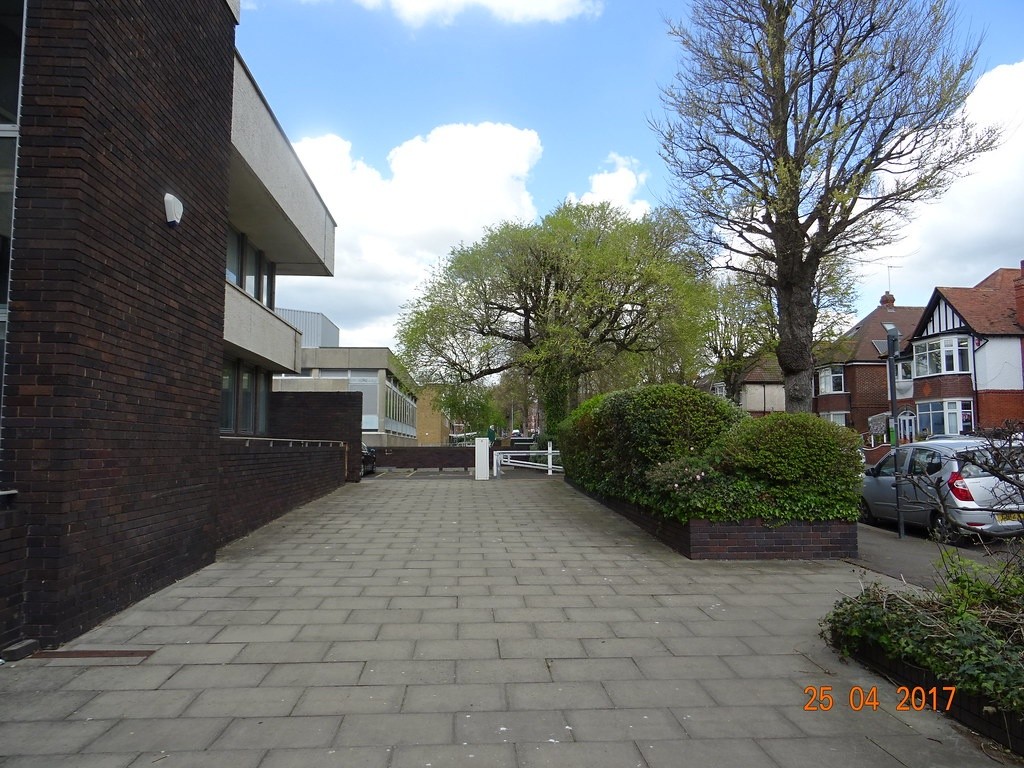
[878,327,908,540]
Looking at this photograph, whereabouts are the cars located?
[512,429,521,436]
[359,441,377,478]
[851,438,1024,548]
